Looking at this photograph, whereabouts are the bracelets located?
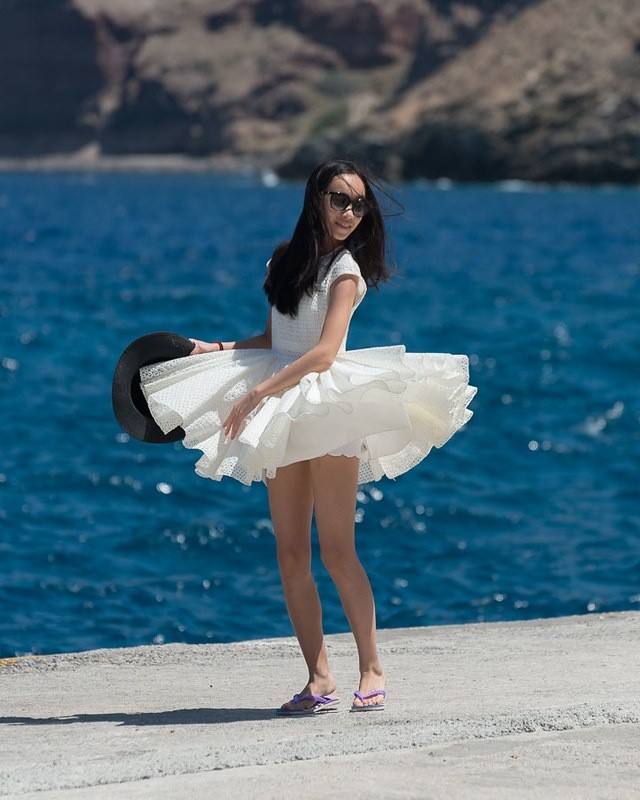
[216,341,225,350]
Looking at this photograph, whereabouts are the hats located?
[112,332,197,443]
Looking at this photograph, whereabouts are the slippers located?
[276,693,341,714]
[351,691,386,711]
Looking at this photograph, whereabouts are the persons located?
[139,159,476,716]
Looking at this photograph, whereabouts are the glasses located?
[322,190,372,217]
